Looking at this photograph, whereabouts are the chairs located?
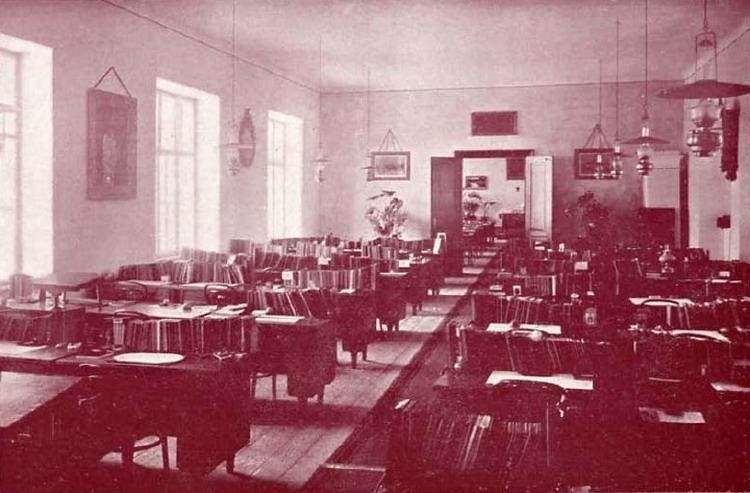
[0,232,750,492]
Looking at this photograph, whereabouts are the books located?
[117,234,443,326]
[389,397,548,492]
[111,313,256,353]
[0,304,85,345]
[444,235,750,381]
[8,271,34,303]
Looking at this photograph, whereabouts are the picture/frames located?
[372,150,410,180]
[574,147,614,180]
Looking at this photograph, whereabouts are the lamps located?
[216,0,258,178]
[625,0,750,178]
